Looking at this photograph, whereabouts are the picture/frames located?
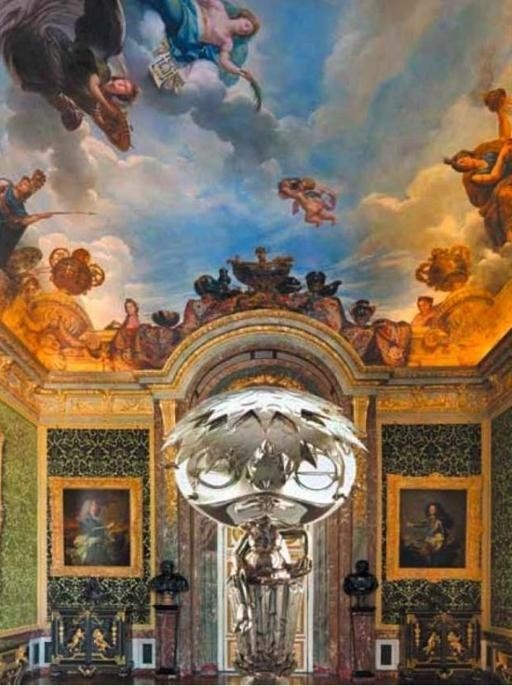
[386,473,484,582]
[47,476,144,579]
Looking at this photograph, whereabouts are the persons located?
[226,515,313,677]
[449,96,512,250]
[400,503,457,571]
[495,649,511,678]
[15,644,28,668]
[148,0,259,95]
[151,561,188,592]
[66,626,86,652]
[422,631,441,656]
[1,167,53,268]
[65,492,128,565]
[343,561,377,611]
[413,296,443,328]
[92,628,110,652]
[0,0,138,132]
[124,299,141,331]
[448,630,467,657]
[279,176,337,227]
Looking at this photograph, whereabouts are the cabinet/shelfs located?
[49,603,133,679]
[399,608,482,681]
